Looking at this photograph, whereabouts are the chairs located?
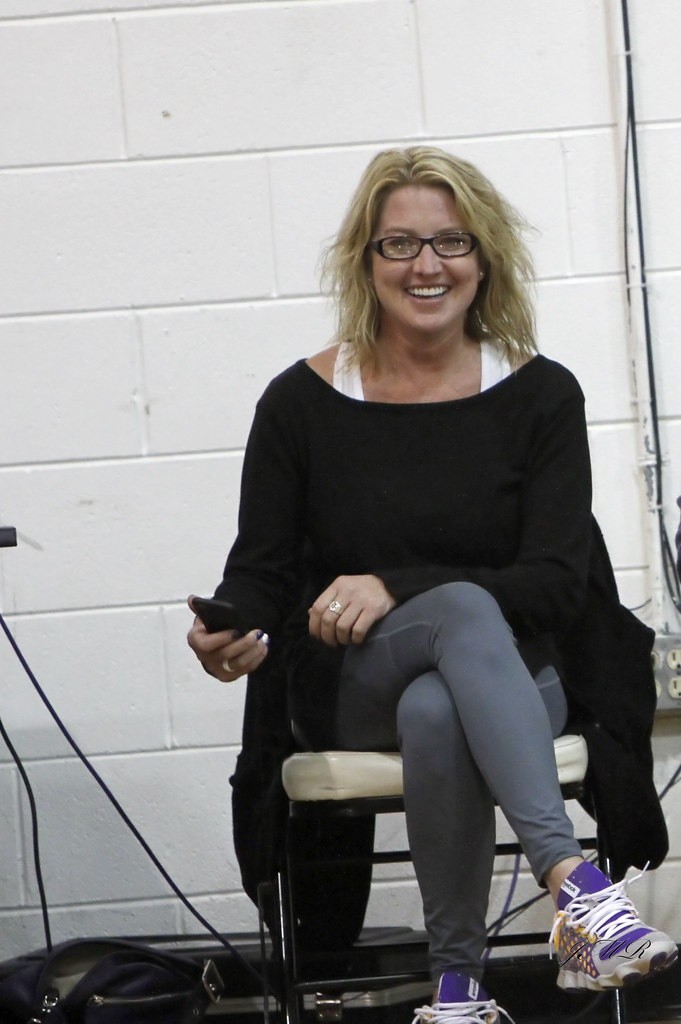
[276,717,621,1024]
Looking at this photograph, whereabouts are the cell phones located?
[192,597,248,637]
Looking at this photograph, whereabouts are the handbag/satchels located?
[1,935,225,1024]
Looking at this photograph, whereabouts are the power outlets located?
[651,634,681,709]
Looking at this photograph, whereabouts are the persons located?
[188,145,680,1024]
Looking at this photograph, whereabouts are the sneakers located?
[548,860,678,991]
[411,972,516,1024]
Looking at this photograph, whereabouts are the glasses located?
[369,232,478,259]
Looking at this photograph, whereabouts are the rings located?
[329,601,344,616]
[223,660,235,672]
